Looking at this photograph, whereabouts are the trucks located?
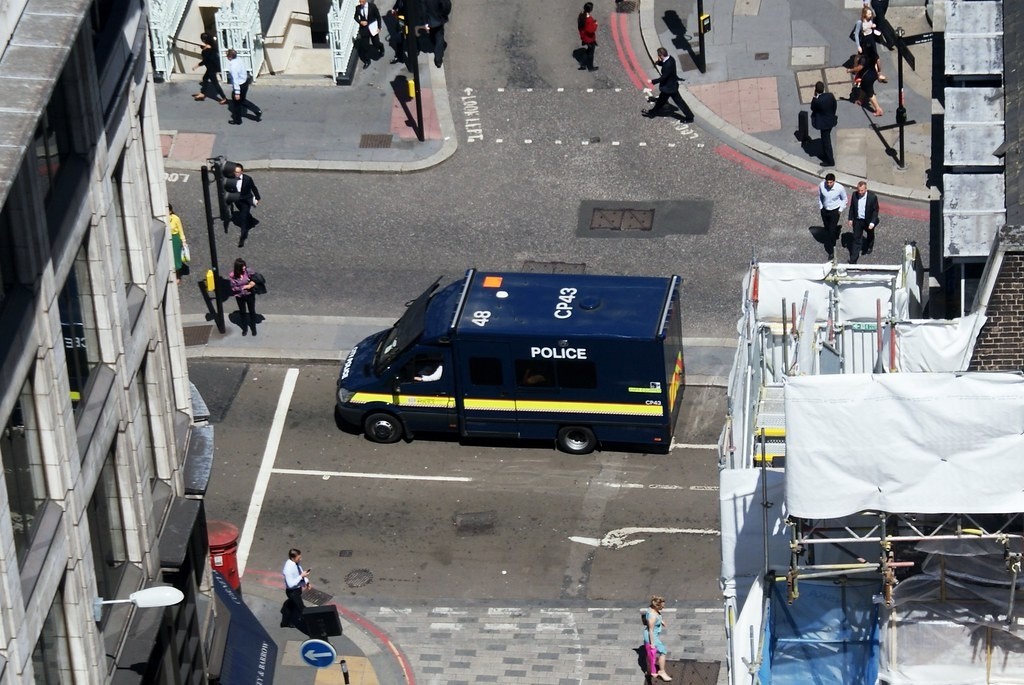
[335,267,686,454]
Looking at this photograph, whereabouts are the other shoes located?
[828,247,872,264]
[588,66,599,71]
[642,108,654,119]
[195,93,229,104]
[390,56,407,64]
[873,108,883,115]
[820,162,834,166]
[242,327,257,336]
[680,118,694,123]
[878,75,886,81]
[363,48,381,69]
[280,622,296,628]
[238,237,245,247]
[645,670,672,681]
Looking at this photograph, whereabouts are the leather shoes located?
[229,110,262,125]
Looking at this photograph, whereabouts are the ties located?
[363,6,365,18]
[296,562,306,587]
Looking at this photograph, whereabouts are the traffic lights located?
[216,158,241,221]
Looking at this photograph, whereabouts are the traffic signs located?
[902,32,934,47]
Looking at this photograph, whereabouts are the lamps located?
[91,586,184,621]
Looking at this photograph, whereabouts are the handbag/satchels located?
[646,642,657,674]
[849,24,864,42]
[640,608,648,624]
[247,73,253,84]
[180,246,191,263]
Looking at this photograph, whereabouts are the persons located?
[168,203,188,286]
[578,2,599,72]
[226,49,263,125]
[810,81,838,166]
[848,180,879,264]
[354,0,385,69]
[231,163,262,248]
[280,548,312,630]
[817,173,848,260]
[846,0,894,116]
[643,595,672,682]
[192,33,228,104]
[390,0,451,69]
[229,258,265,336]
[641,47,695,124]
